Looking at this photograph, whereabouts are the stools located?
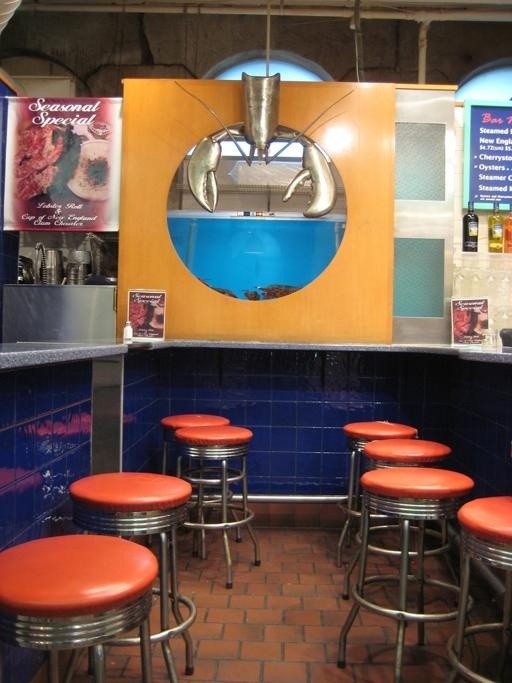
[337,467,481,683]
[446,496,512,683]
[337,419,426,568]
[64,472,197,682]
[342,438,462,600]
[175,426,261,589]
[0,535,159,683]
[147,415,242,558]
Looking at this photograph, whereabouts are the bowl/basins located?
[39,247,64,285]
[65,251,92,284]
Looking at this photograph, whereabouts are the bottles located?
[123,320,133,344]
[463,200,479,251]
[504,211,512,253]
[489,201,504,252]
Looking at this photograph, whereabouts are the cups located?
[480,328,498,349]
